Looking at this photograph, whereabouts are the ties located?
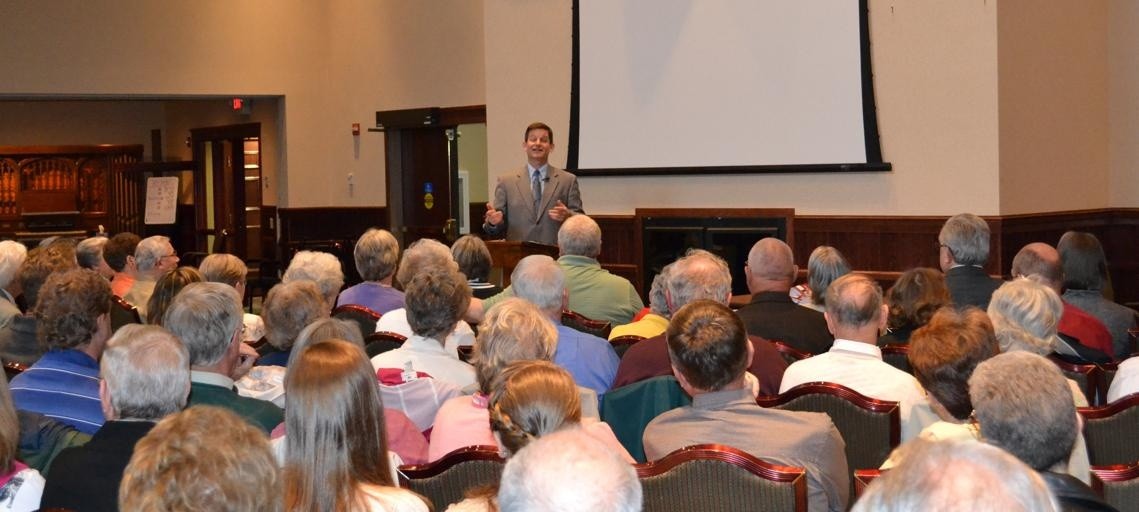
[531,169,543,215]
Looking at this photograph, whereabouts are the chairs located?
[1,234,1139,512]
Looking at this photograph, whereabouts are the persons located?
[482,121,585,245]
[0,215,1139,510]
